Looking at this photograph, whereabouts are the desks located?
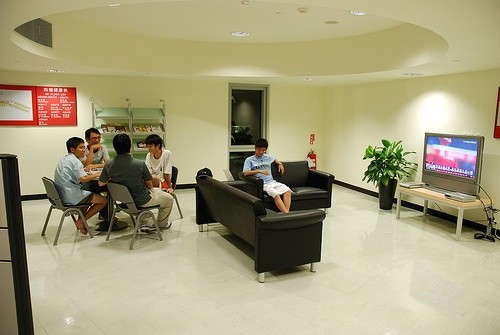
[397,186,495,237]
[88,170,160,232]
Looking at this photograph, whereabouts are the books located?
[101,123,129,133]
[133,125,162,132]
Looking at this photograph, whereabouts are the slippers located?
[75,220,87,234]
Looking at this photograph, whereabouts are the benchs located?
[239,161,335,212]
[195,175,326,284]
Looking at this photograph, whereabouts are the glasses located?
[89,137,101,140]
[256,147,266,153]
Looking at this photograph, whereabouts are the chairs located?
[40,177,94,246]
[105,182,162,250]
[167,166,183,219]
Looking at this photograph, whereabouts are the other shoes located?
[105,217,117,222]
[141,222,157,232]
[153,221,172,229]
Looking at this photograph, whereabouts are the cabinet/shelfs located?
[91,97,167,162]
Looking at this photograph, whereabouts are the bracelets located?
[277,162,282,165]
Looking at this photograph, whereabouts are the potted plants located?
[362,139,418,210]
[231,126,253,157]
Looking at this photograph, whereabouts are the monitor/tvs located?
[421,132,484,196]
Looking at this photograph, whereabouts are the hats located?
[197,168,212,179]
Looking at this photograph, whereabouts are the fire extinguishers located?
[308,150,316,170]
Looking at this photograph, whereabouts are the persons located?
[243,138,291,214]
[54,137,108,235]
[78,128,110,189]
[98,133,173,231]
[145,134,173,193]
